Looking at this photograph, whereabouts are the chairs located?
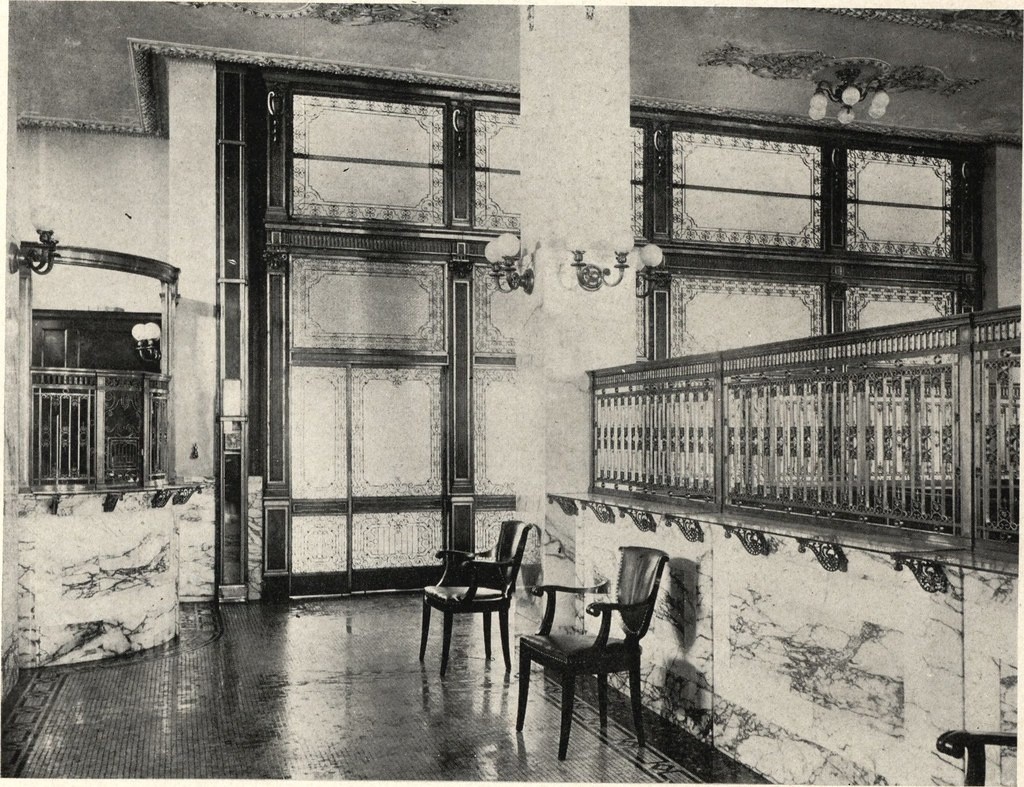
[419,520,534,677]
[516,546,669,761]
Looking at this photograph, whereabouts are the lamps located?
[7,204,64,275]
[808,68,890,125]
[565,226,634,292]
[633,244,664,298]
[132,322,166,363]
[485,233,534,294]
